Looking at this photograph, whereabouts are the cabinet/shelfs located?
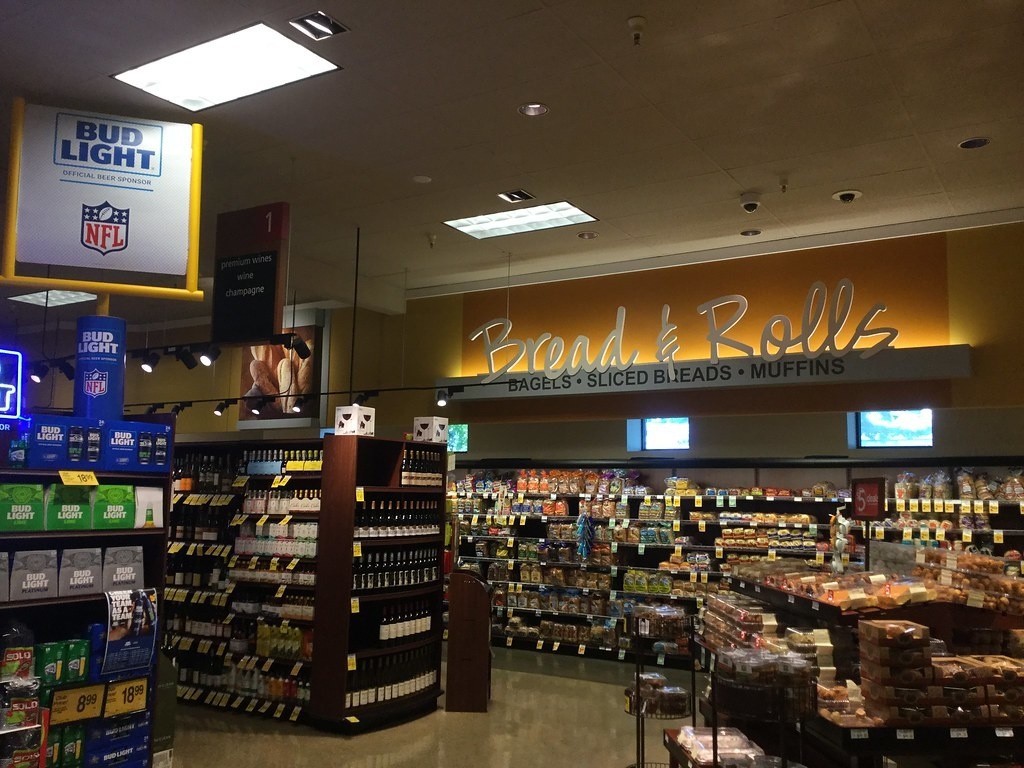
[448,569,494,713]
[444,456,1024,673]
[165,436,448,741]
[0,413,177,768]
[697,575,1024,768]
[624,616,696,768]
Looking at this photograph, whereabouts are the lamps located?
[292,395,315,413]
[213,400,238,416]
[146,403,164,414]
[251,397,276,414]
[27,362,50,383]
[130,350,160,372]
[293,336,311,359]
[171,402,192,417]
[437,387,464,406]
[163,347,198,369]
[352,391,379,405]
[190,344,221,366]
[49,359,74,381]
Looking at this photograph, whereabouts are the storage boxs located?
[956,654,1024,685]
[33,616,159,768]
[859,643,932,666]
[986,685,1024,705]
[0,483,44,532]
[937,685,985,705]
[57,547,103,597]
[989,704,1024,724]
[862,701,941,725]
[859,663,933,687]
[91,484,135,530]
[9,549,58,601]
[133,485,164,529]
[858,618,930,645]
[940,705,990,724]
[43,483,91,530]
[103,546,145,592]
[860,676,937,704]
[0,552,9,602]
[697,592,865,714]
[932,656,988,686]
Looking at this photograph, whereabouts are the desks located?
[662,729,769,768]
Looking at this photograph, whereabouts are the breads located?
[766,549,1024,616]
[704,597,884,727]
